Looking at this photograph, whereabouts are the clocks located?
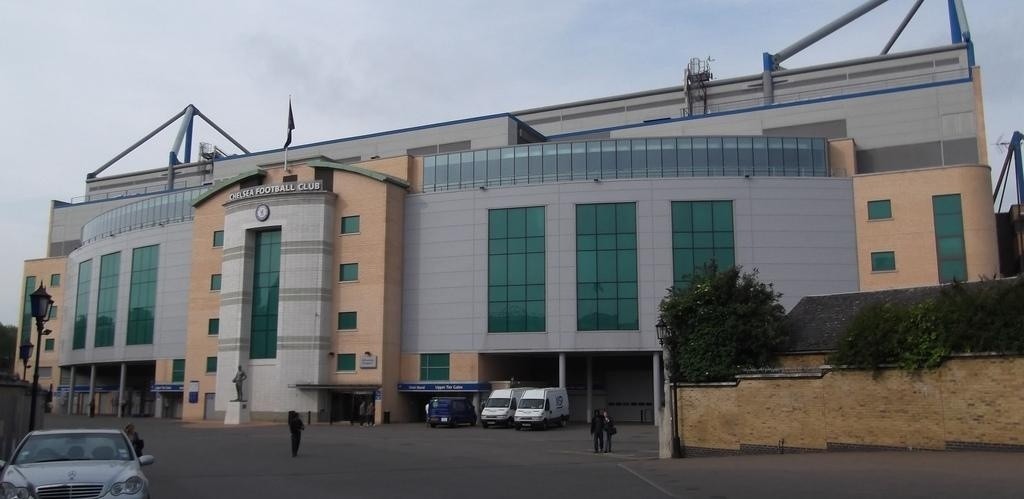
[255,203,269,221]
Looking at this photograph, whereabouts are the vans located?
[480,387,540,429]
[428,396,477,428]
[513,387,571,431]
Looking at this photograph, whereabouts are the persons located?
[366,401,375,427]
[123,424,144,466]
[289,413,304,458]
[601,410,613,452]
[232,365,247,401]
[359,401,366,428]
[590,410,611,453]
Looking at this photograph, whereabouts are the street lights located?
[18,279,54,429]
[656,318,684,457]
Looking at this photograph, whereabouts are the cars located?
[1,428,155,499]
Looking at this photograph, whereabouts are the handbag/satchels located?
[608,428,616,434]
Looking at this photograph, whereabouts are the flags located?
[283,98,295,149]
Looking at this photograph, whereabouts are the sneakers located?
[594,448,611,453]
